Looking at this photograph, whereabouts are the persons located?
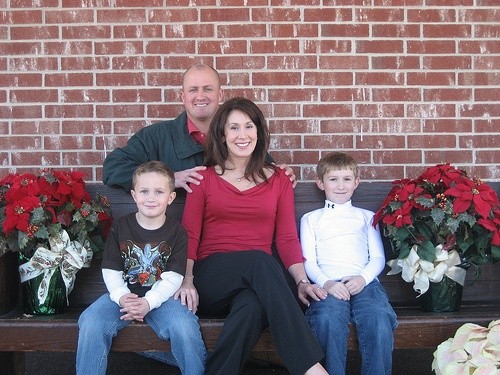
[102,63,297,193]
[300,152,397,375]
[174,97,328,375]
[76,160,208,375]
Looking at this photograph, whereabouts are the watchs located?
[297,280,310,287]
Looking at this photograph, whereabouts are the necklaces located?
[232,171,246,181]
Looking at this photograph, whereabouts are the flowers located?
[0,165,112,268]
[370,161,500,294]
[429,317,500,375]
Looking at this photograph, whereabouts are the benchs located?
[0,180,500,353]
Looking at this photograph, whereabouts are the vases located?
[19,250,73,316]
[423,264,469,310]
